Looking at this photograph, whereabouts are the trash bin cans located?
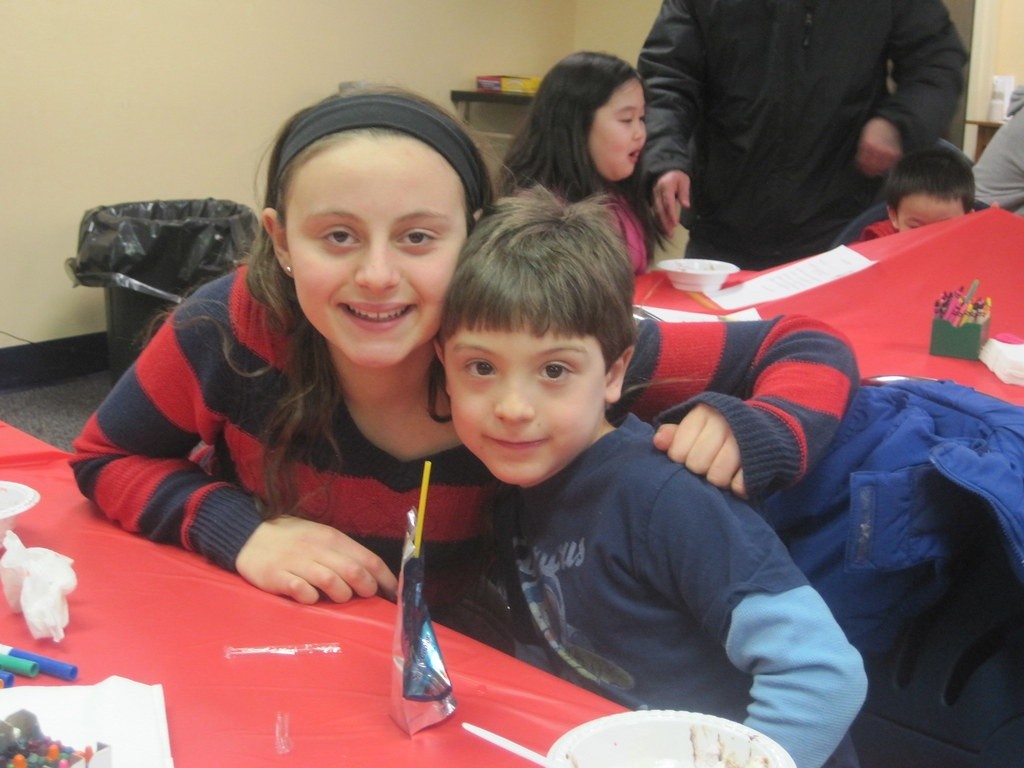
[92,199,262,386]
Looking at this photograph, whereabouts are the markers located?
[0,645,79,689]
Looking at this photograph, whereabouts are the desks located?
[0,420,632,768]
[631,209,1024,420]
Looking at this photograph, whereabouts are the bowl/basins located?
[0,481,41,540]
[657,257,740,292]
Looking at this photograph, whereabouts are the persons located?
[65,0,1024,768]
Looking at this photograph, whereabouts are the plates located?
[544,709,798,768]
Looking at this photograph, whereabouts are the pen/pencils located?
[933,279,992,326]
